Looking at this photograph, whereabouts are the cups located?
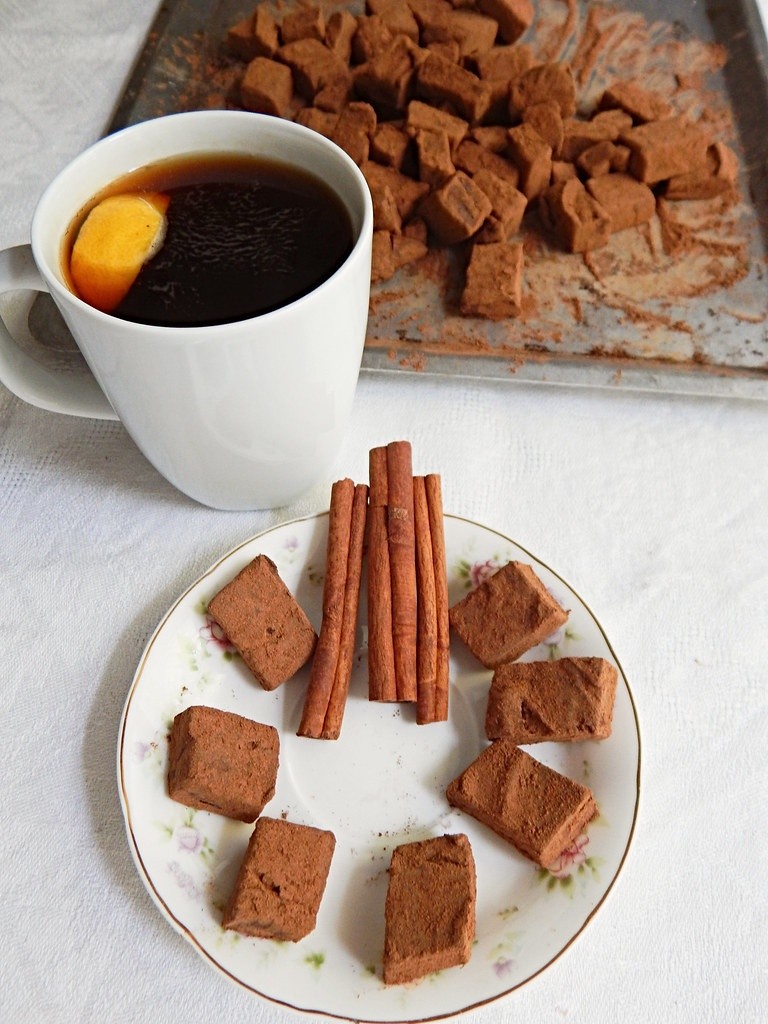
[0,111,373,512]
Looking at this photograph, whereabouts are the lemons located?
[69,189,170,313]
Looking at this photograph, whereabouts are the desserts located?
[226,1,738,317]
[164,441,618,983]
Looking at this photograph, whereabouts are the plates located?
[115,504,642,1024]
[26,1,768,405]
